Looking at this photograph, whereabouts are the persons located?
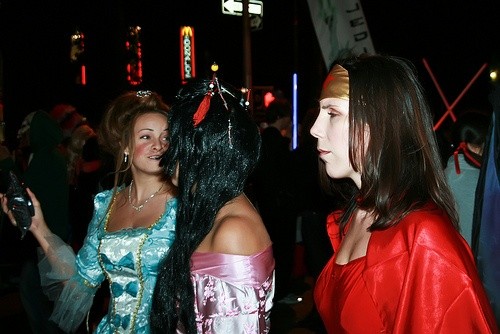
[310,53,500,334]
[0,76,500,334]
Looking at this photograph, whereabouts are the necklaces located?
[128,179,165,212]
